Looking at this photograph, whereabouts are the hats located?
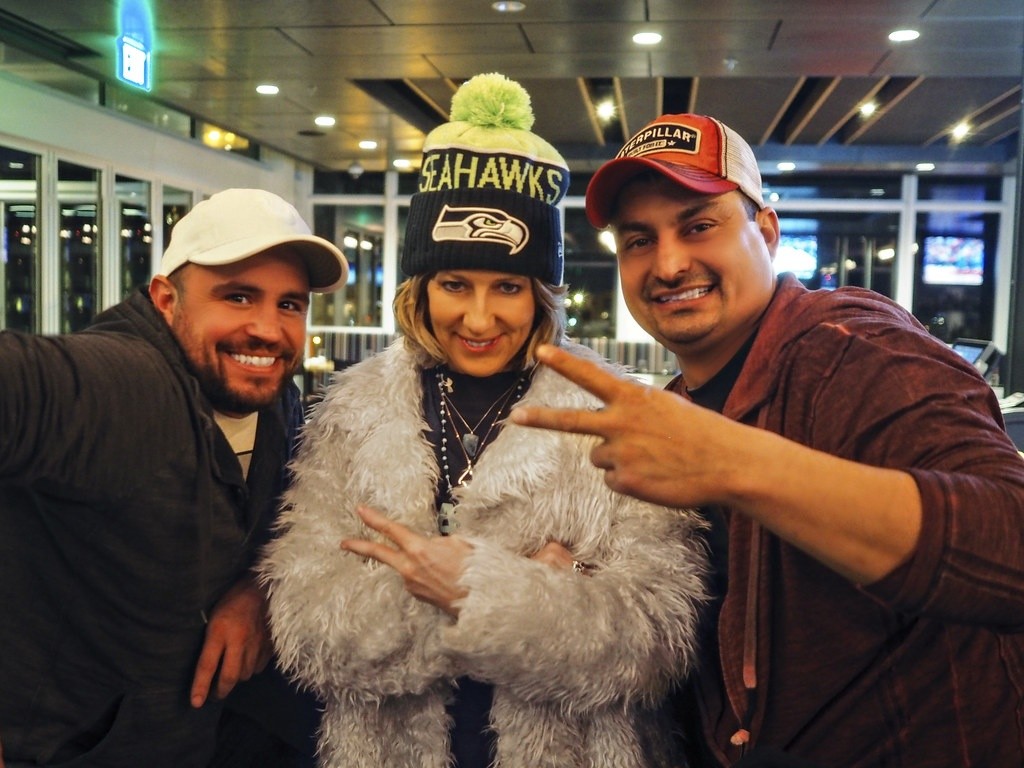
[157,188,349,293]
[585,113,765,229]
[401,72,569,288]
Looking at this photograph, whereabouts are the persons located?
[268,76,712,768]
[511,120,1024,768]
[0,184,349,767]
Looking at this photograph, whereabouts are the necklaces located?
[432,362,542,539]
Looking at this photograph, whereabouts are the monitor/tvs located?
[919,234,990,287]
[951,337,1001,378]
[770,232,820,283]
